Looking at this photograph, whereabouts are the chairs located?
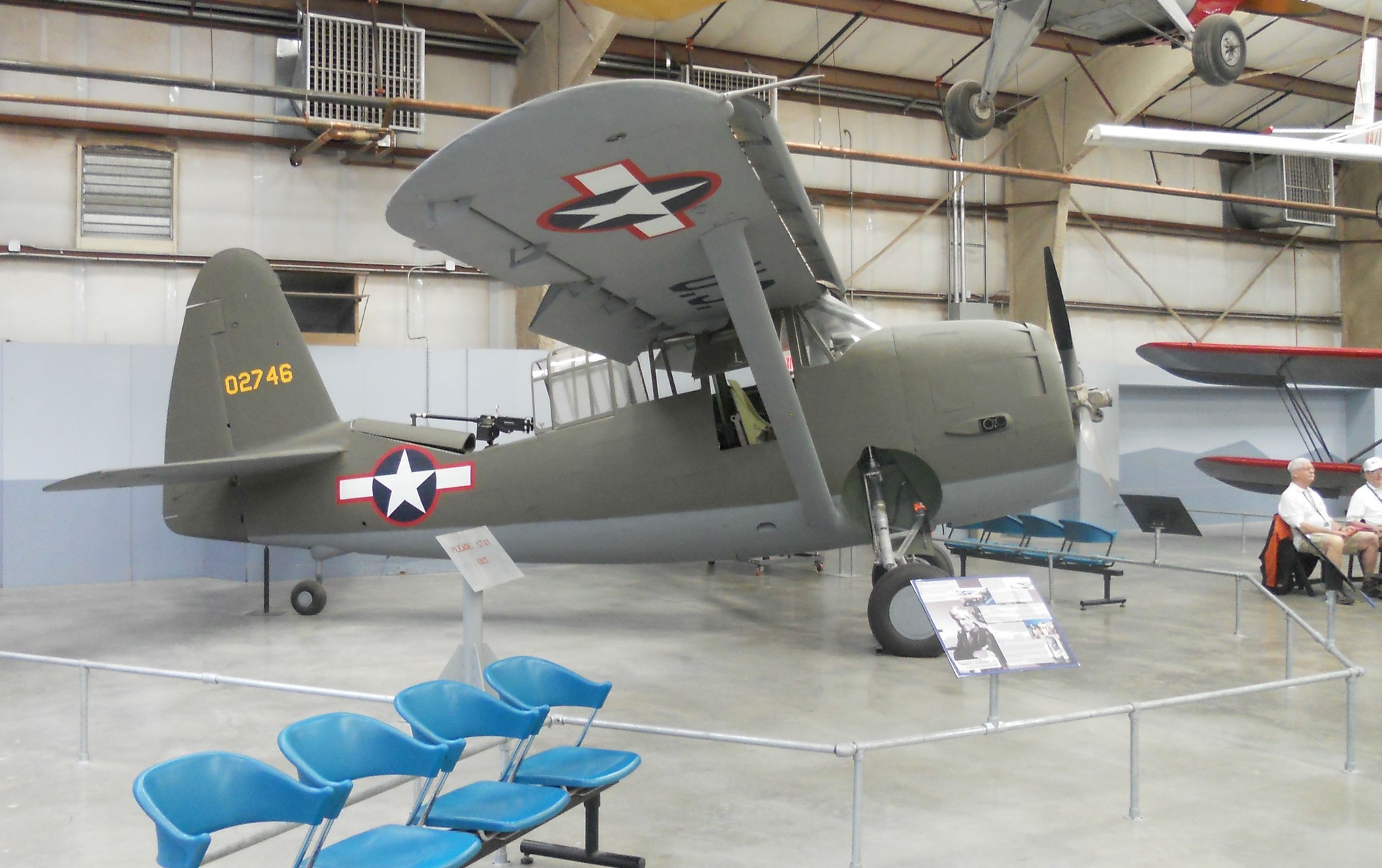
[728,379,773,445]
[1258,510,1382,597]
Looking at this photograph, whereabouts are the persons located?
[1277,457,1382,604]
[1346,457,1382,551]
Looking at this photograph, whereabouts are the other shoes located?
[1326,589,1354,605]
[1361,578,1382,598]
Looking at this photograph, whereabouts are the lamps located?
[1084,77,1382,164]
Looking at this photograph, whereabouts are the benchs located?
[134,655,647,868]
[944,513,1127,610]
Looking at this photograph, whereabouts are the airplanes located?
[45,73,1114,658]
[944,1,1382,499]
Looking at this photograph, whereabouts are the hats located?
[1363,457,1382,471]
